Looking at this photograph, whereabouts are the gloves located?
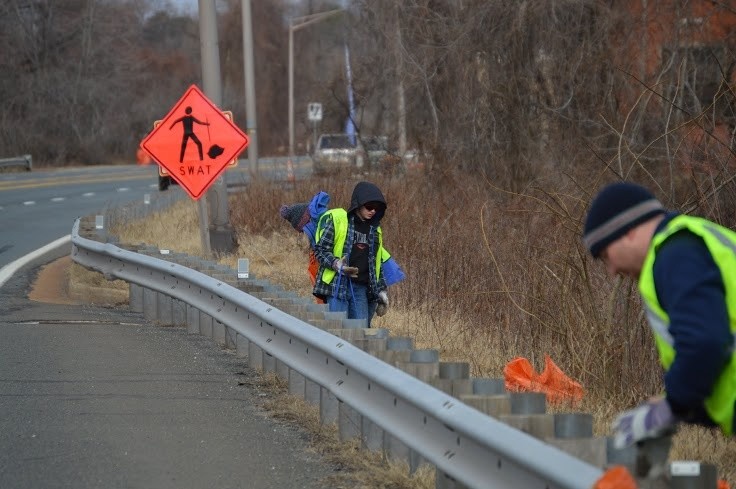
[611,399,679,450]
[333,256,358,278]
[376,291,389,316]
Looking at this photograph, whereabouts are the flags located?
[344,42,357,147]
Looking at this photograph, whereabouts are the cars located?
[364,136,388,166]
[311,133,368,174]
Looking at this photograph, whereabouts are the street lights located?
[288,5,360,190]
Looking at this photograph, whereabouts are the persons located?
[313,182,388,328]
[582,181,736,450]
[280,192,404,304]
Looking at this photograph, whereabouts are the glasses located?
[364,203,377,211]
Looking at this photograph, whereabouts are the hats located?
[280,203,311,234]
[582,181,665,259]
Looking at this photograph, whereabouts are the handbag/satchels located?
[329,264,360,320]
